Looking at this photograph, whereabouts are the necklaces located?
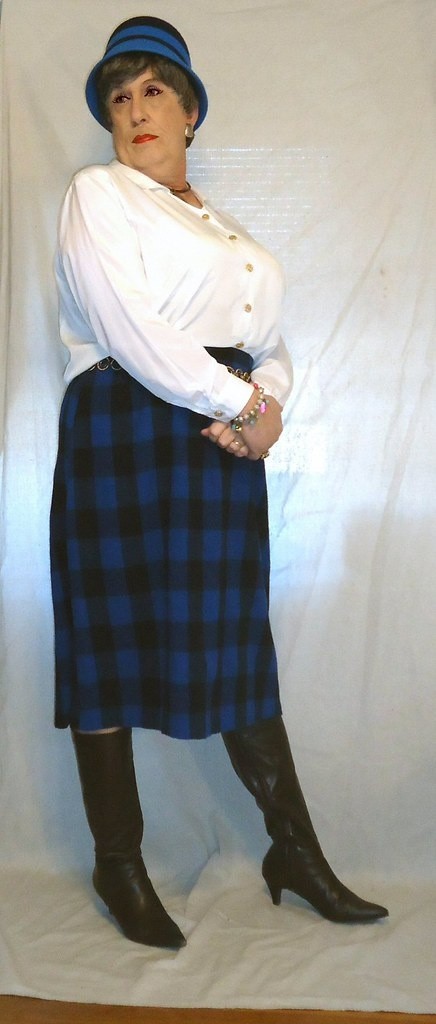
[168,181,191,193]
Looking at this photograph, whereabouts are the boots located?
[216,715,389,924]
[67,726,187,948]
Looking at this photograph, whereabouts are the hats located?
[85,15,208,149]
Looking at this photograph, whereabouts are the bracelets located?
[231,384,269,430]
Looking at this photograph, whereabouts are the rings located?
[261,452,269,459]
[233,440,242,448]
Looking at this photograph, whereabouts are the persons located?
[48,15,389,949]
